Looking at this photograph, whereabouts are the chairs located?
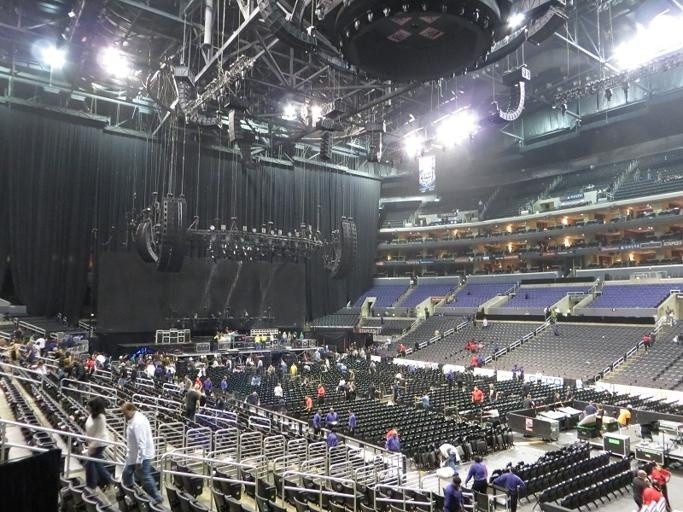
[382,157,681,231]
[307,280,681,351]
[0,351,682,511]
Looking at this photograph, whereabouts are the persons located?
[79,397,111,491]
[631,469,648,508]
[0,167,682,450]
[442,476,465,511]
[433,442,460,475]
[641,477,665,511]
[648,462,671,512]
[119,401,164,506]
[464,454,488,498]
[491,466,525,511]
